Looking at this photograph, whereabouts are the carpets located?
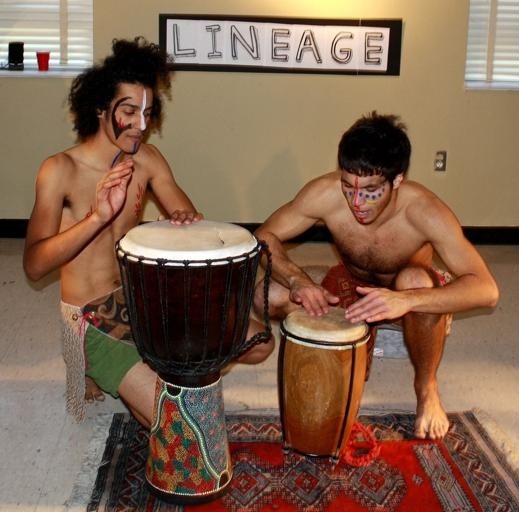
[64,401,519,512]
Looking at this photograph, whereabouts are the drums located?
[115,220,262,506]
[277,307,372,464]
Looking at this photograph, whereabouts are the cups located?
[36,51,49,71]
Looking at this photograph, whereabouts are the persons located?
[22,35,275,432]
[250,110,501,443]
